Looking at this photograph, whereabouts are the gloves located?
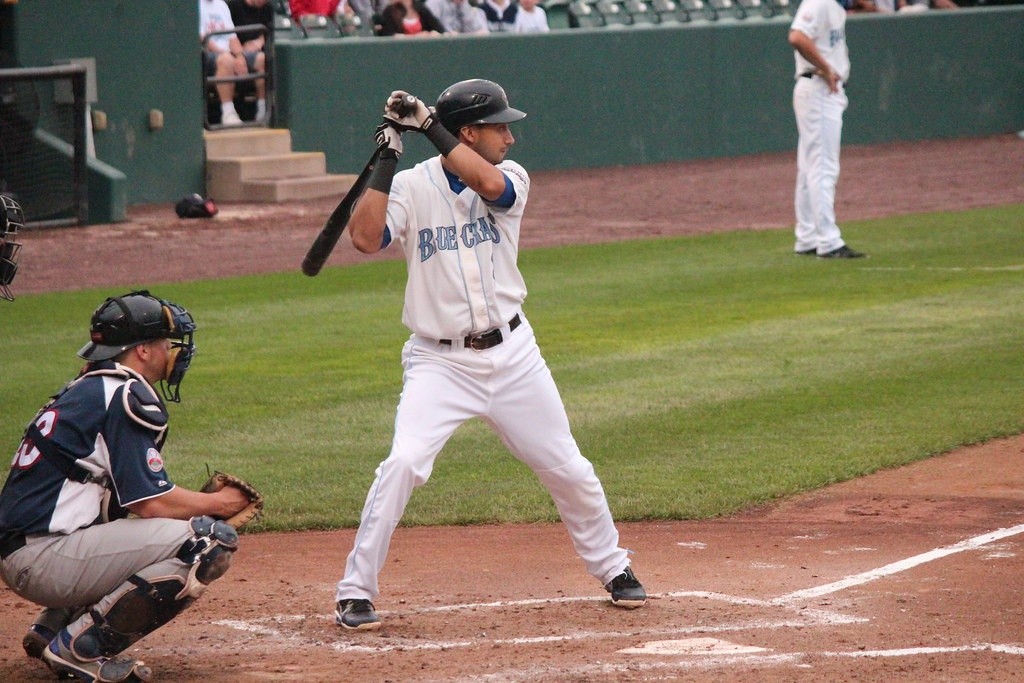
[382,89,439,131]
[374,120,403,160]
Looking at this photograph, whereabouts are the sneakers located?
[333,598,384,633]
[21,625,60,669]
[40,630,148,683]
[603,565,648,609]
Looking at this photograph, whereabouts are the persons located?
[787,0,869,258]
[0,291,264,683]
[287,0,550,37]
[199,0,275,128]
[333,77,648,630]
[843,0,959,13]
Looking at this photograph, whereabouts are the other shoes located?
[256,110,264,123]
[794,248,821,256]
[817,244,870,261]
[221,108,245,126]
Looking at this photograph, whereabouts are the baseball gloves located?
[197,461,266,534]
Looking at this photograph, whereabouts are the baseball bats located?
[300,93,417,278]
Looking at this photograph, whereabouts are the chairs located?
[270,0,797,40]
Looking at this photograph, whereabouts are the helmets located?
[76,292,171,364]
[436,77,528,137]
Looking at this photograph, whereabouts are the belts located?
[803,72,848,91]
[437,313,522,352]
